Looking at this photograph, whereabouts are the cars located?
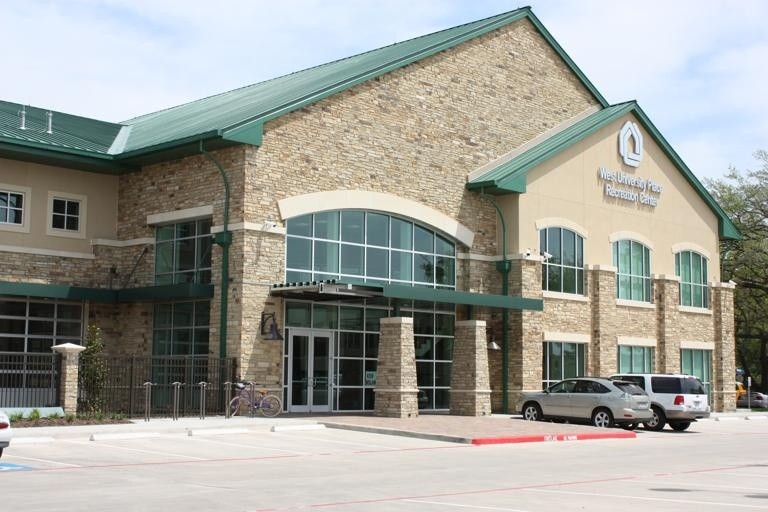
[736,392,768,408]
[417,387,429,409]
[0,411,13,459]
[514,374,654,431]
[735,381,748,401]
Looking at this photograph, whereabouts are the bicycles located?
[226,377,283,420]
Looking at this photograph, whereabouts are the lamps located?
[261,312,284,340]
[486,324,501,350]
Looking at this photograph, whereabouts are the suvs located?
[609,373,711,431]
